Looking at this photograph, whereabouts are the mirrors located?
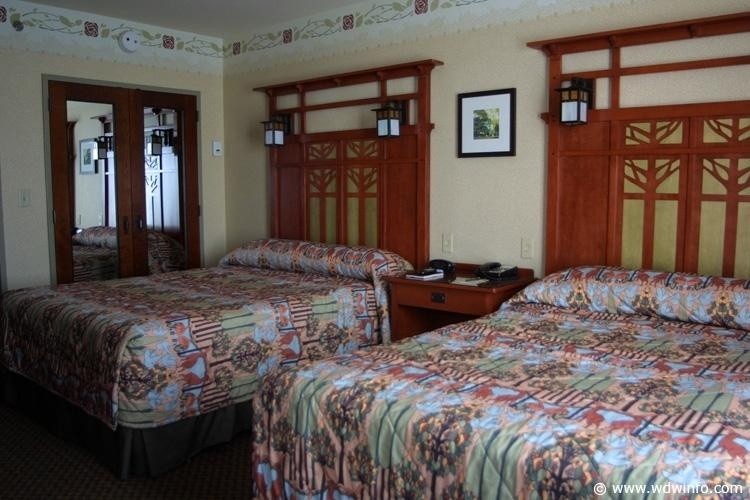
[66,99,189,281]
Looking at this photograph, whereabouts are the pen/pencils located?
[465,278,480,282]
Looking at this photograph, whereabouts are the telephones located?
[474,262,519,281]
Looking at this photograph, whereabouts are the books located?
[405,272,443,278]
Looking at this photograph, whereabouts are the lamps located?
[259,111,293,148]
[369,98,409,138]
[552,76,595,127]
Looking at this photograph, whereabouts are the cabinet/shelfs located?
[47,78,201,285]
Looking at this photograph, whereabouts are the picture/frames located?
[456,88,517,159]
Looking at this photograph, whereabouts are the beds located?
[0,237,749,500]
